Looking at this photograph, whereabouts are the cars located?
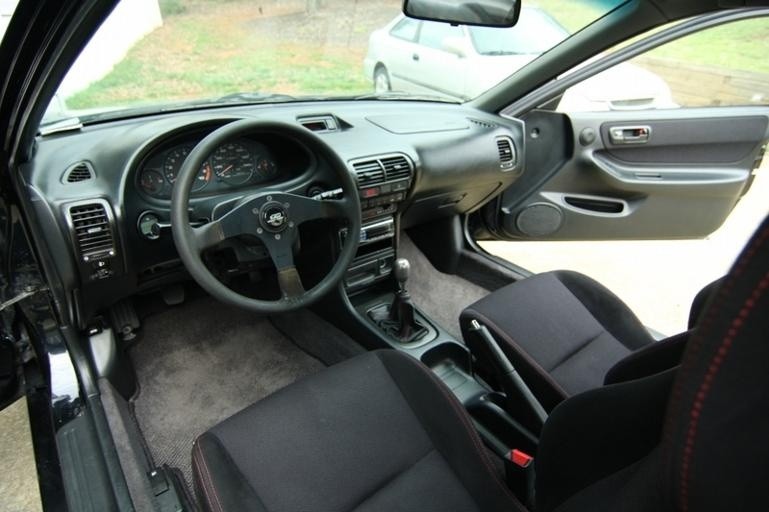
[362,2,678,114]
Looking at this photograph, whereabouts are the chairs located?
[459,269,721,434]
[192,214,769,512]
